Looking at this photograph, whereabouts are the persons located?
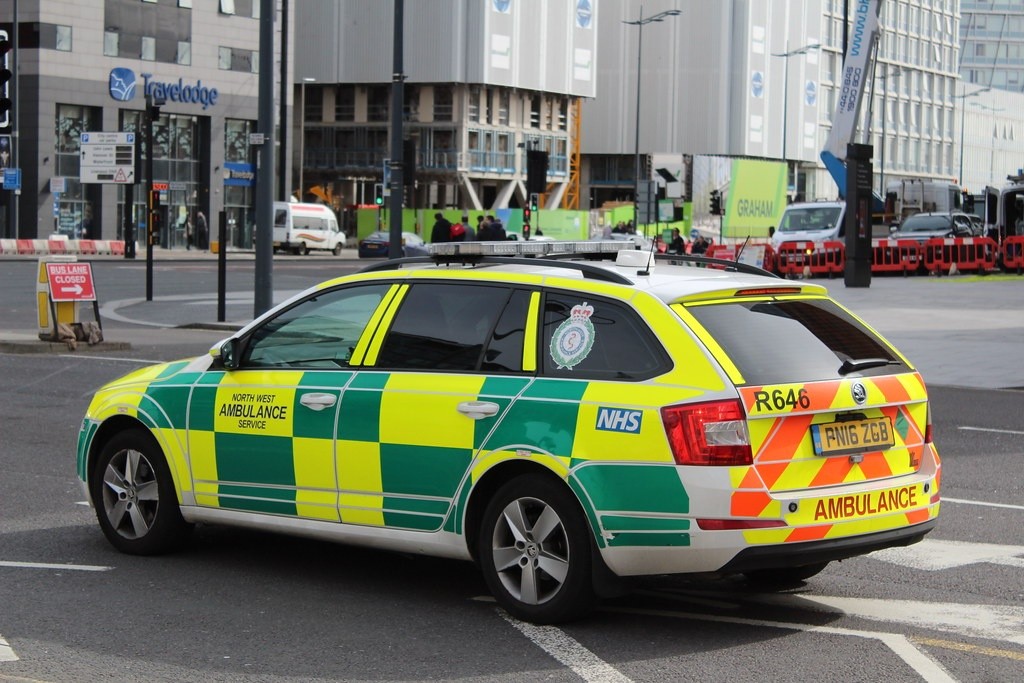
[186,212,208,249]
[669,228,685,255]
[692,237,709,255]
[431,213,507,243]
[612,220,633,234]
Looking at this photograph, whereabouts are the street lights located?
[622,5,683,228]
[771,41,820,158]
[299,77,316,203]
[879,61,906,195]
[953,82,990,186]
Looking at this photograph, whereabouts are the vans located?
[273,201,346,257]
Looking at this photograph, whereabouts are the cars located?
[358,232,429,259]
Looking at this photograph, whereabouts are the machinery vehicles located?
[770,0,964,269]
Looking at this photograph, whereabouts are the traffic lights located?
[530,194,537,211]
[523,225,530,237]
[0,29,13,128]
[374,183,384,206]
[524,209,530,222]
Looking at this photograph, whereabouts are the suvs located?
[888,210,974,265]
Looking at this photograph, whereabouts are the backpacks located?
[451,222,466,242]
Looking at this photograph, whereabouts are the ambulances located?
[76,240,941,623]
